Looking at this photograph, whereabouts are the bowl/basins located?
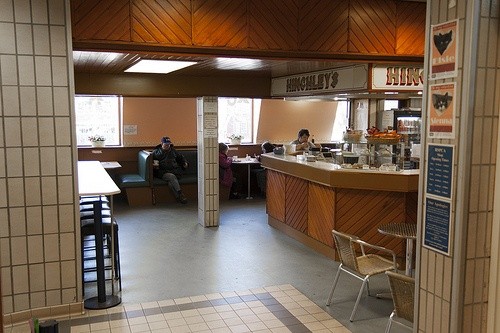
[296,155,304,160]
[351,133,363,143]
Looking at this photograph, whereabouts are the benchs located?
[151,148,198,201]
[118,150,153,208]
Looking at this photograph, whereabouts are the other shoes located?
[178,191,188,203]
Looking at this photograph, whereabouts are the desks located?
[76,161,121,310]
[230,157,261,199]
[375,222,417,299]
[102,161,122,168]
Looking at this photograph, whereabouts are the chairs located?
[384,271,415,333]
[326,230,400,322]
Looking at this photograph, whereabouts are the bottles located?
[343,141,349,151]
[398,119,420,141]
[304,152,309,161]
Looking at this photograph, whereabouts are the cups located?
[233,156,238,159]
[335,151,342,165]
[324,152,332,157]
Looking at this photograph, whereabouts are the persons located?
[151,137,188,203]
[219,144,239,200]
[251,142,275,196]
[290,128,317,150]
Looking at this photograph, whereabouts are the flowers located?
[88,135,106,142]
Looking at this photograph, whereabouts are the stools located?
[78,195,122,296]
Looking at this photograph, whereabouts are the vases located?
[92,141,105,146]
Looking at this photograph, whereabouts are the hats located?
[161,136,172,144]
